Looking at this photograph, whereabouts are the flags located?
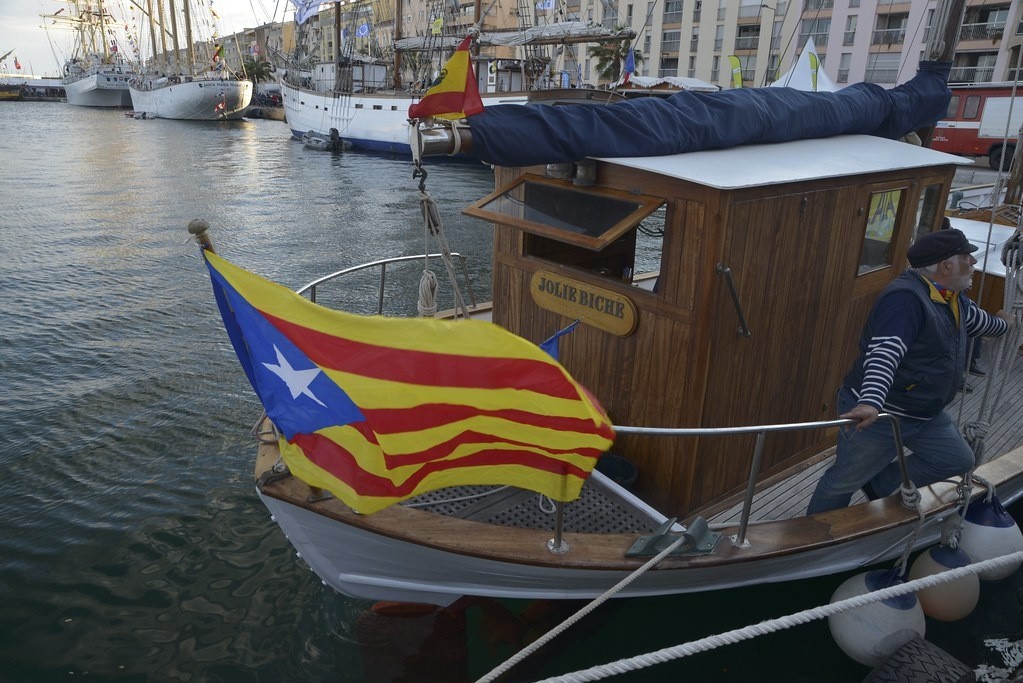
[197,247,616,516]
[354,23,370,37]
[431,18,443,34]
[408,34,485,121]
[211,41,225,113]
[621,46,635,84]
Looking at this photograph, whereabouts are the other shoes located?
[863,483,878,502]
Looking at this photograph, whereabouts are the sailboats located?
[125,0,255,121]
[190,0,1022,673]
[263,0,638,164]
[38,0,143,108]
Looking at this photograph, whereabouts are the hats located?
[908,228,979,268]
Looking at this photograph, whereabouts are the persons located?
[806,227,1015,517]
